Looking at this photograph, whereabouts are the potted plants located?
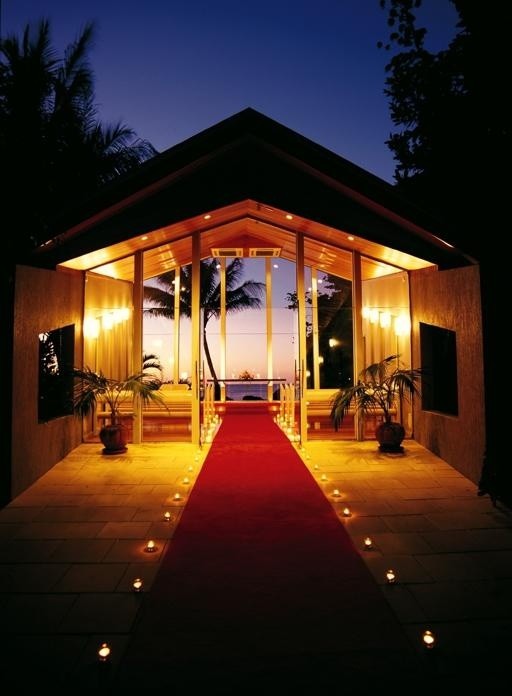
[330,344,429,456]
[70,351,174,456]
